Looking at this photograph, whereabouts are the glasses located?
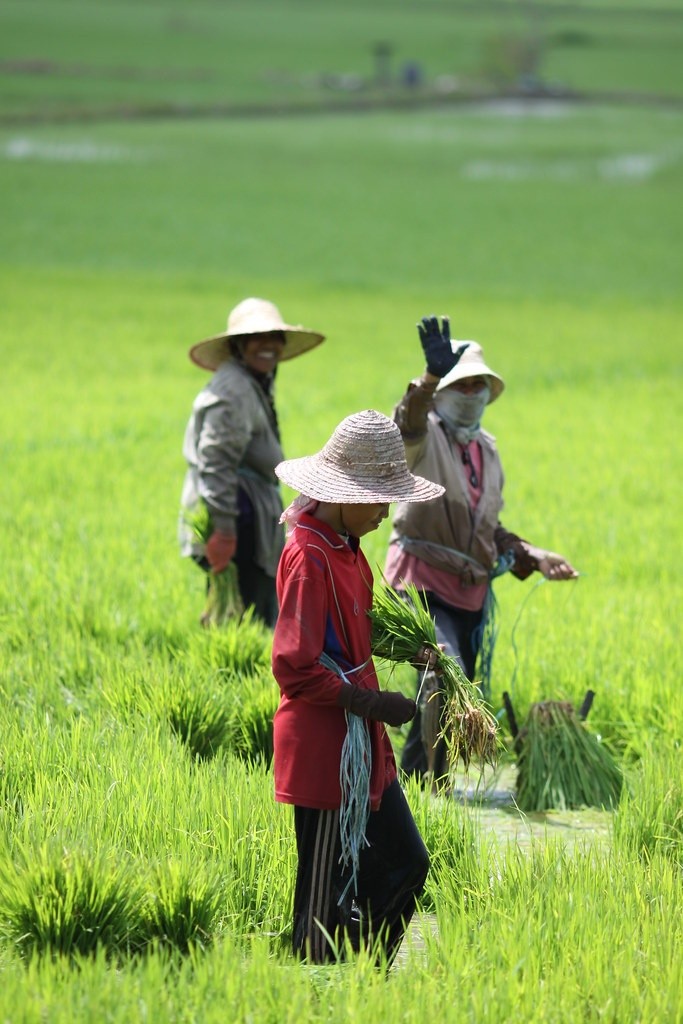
[461,448,478,487]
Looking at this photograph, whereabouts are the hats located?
[434,340,505,406]
[189,297,325,370]
[274,409,446,504]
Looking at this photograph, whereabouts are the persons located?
[382,315,580,800]
[177,299,327,628]
[272,410,446,982]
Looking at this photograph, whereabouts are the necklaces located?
[461,450,478,486]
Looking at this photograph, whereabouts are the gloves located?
[206,532,236,573]
[417,316,470,379]
